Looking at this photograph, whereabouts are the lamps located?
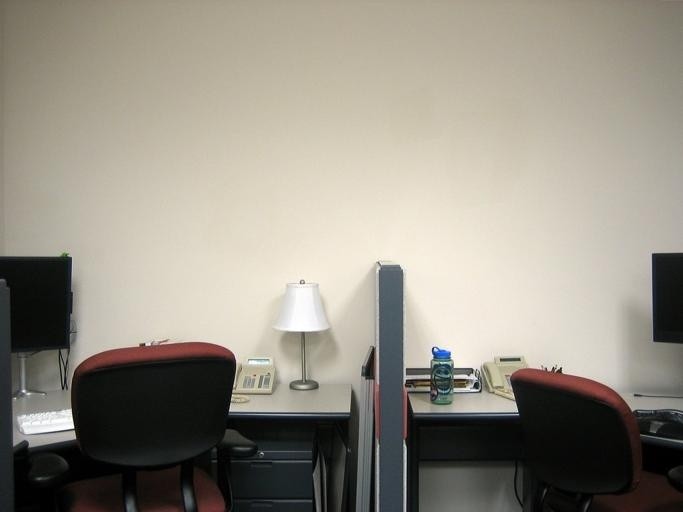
[271,280,329,390]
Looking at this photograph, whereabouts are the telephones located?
[232,355,276,394]
[481,355,529,393]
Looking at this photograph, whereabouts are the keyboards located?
[632,408,683,439]
[17,409,75,434]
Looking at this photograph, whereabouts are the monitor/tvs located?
[651,253,682,344]
[0,255,73,400]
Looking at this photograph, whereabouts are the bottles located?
[429,347,455,405]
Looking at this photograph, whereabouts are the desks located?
[9,379,353,511]
[405,387,682,511]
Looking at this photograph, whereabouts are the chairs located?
[24,340,257,511]
[509,368,682,512]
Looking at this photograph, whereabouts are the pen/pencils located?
[542,365,563,374]
